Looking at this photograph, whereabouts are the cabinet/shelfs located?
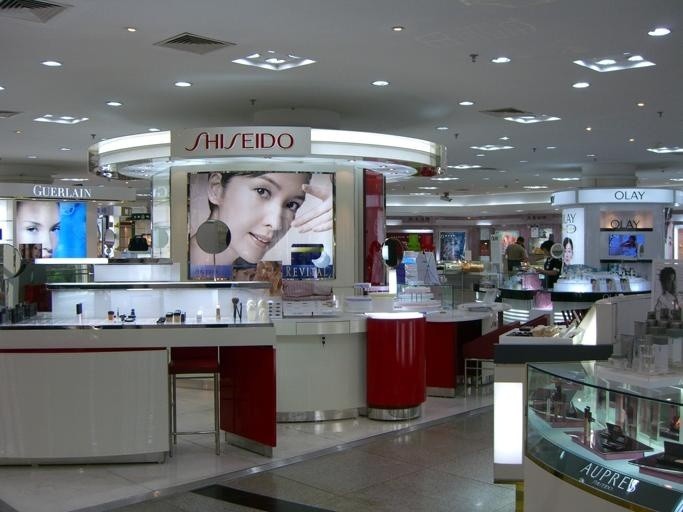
[473,281,652,328]
[522,361,683,512]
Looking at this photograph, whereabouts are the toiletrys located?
[156,308,186,325]
[106,306,139,324]
[606,308,683,378]
[75,303,83,324]
[195,311,203,322]
[216,306,221,322]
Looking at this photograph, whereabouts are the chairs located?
[168,346,221,457]
[520,313,547,326]
[463,320,520,400]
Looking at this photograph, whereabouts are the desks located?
[425,301,512,399]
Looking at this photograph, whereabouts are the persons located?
[16,198,60,258]
[535,241,563,288]
[194,173,334,277]
[505,237,529,272]
[620,235,639,256]
[232,257,257,281]
[562,238,575,267]
[655,267,683,308]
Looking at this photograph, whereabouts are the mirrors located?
[0,244,23,309]
[381,238,404,269]
[550,242,568,278]
[104,229,115,258]
[196,219,231,282]
